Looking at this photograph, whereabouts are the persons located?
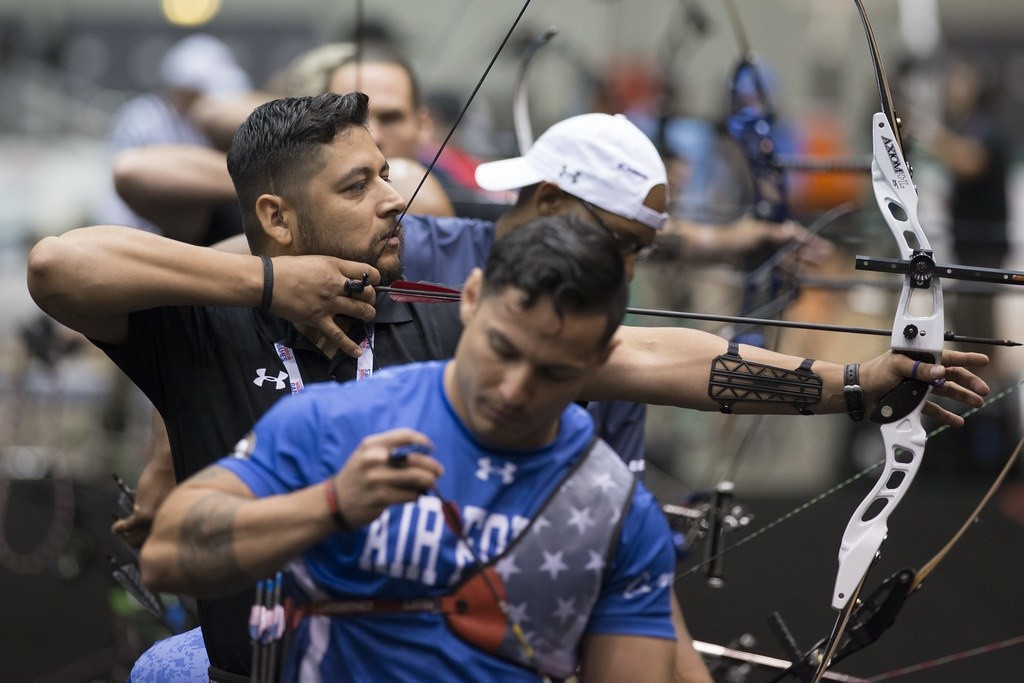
[26,32,1013,683]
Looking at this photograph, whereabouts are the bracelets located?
[253,254,274,315]
[324,477,349,531]
[843,365,866,423]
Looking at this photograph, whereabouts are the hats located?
[474,112,670,231]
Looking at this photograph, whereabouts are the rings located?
[343,280,365,299]
[390,444,408,468]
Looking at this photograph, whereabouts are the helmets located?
[620,111,759,226]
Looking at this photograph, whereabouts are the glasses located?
[580,199,660,266]
[370,107,417,127]
